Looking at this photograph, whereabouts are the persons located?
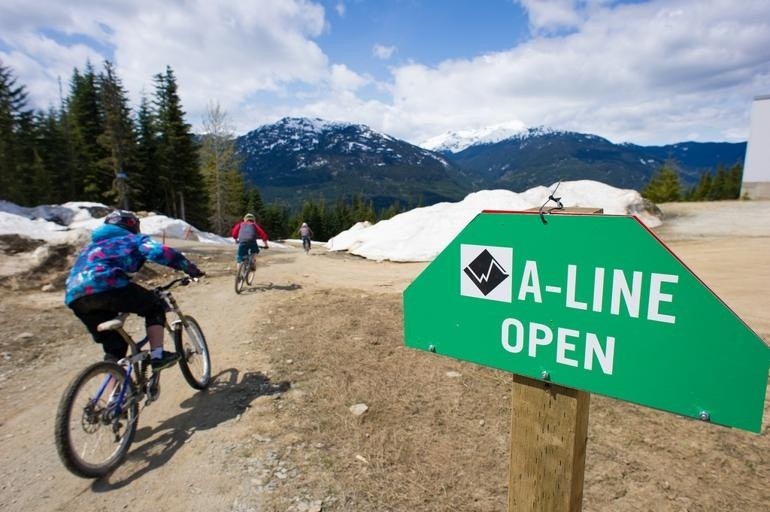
[231,211,270,283]
[64,209,205,417]
[296,219,315,249]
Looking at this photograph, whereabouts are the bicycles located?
[54,270,211,480]
[233,240,269,295]
[298,234,315,254]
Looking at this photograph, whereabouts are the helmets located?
[302,223,307,227]
[244,213,255,222]
[104,208,141,230]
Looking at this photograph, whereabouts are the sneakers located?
[150,349,183,373]
[237,263,258,283]
[107,400,129,419]
